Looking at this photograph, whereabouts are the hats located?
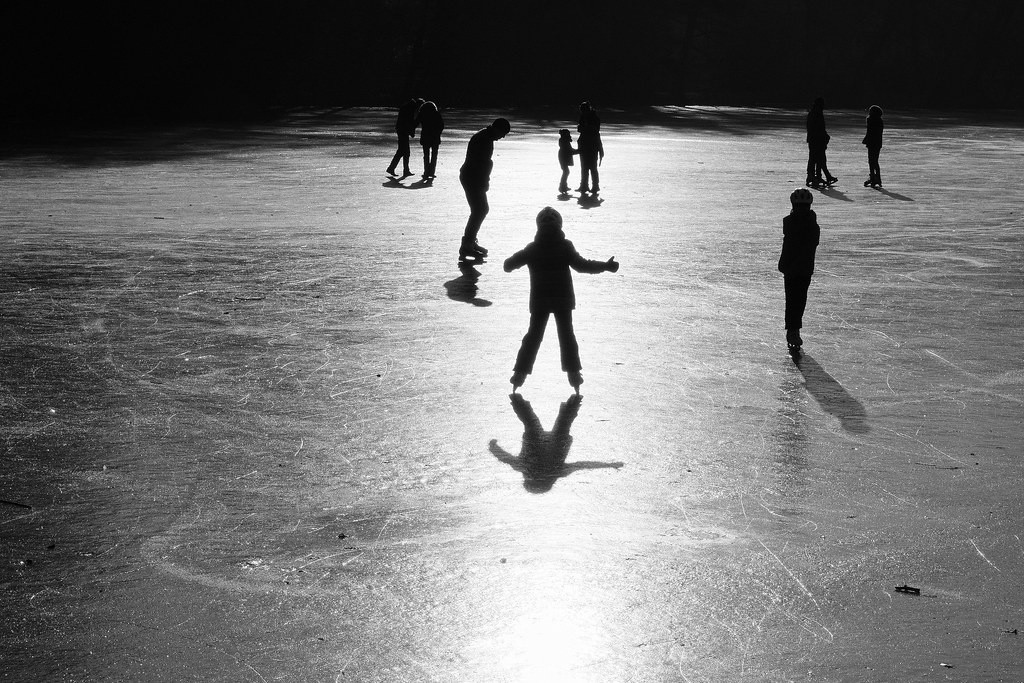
[790,189,813,204]
[536,207,561,223]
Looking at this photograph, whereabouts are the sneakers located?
[510,372,526,387]
[459,237,487,257]
[787,329,803,346]
[473,239,487,254]
[805,176,839,190]
[568,376,584,387]
[558,183,572,195]
[862,176,882,188]
[574,185,600,196]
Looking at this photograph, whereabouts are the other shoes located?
[422,171,437,181]
[387,168,399,176]
[403,171,415,176]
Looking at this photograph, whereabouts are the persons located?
[387,98,444,178]
[505,207,619,396]
[778,188,820,349]
[560,101,603,193]
[460,117,511,257]
[862,105,884,187]
[806,97,837,187]
